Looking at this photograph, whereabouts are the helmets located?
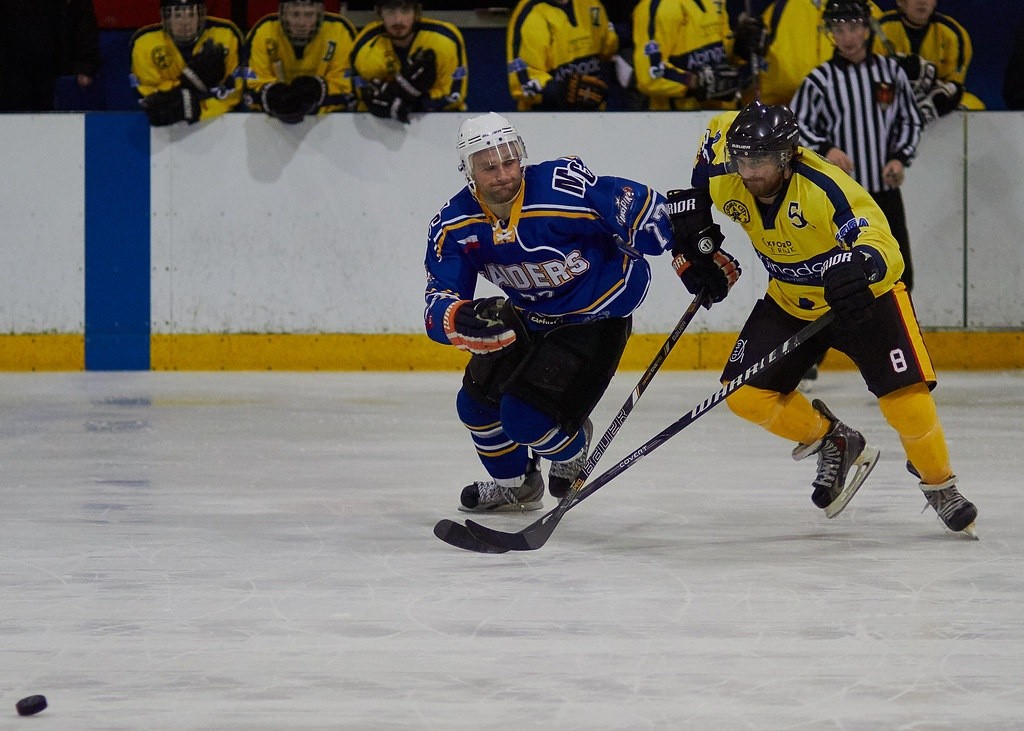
[727,100,799,156]
[819,1,870,30]
[454,111,518,177]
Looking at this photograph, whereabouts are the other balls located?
[16,695,47,716]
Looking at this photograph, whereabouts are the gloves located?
[732,18,770,60]
[364,47,437,125]
[695,55,740,102]
[898,53,964,121]
[258,74,328,124]
[551,53,616,112]
[670,249,742,310]
[140,88,201,127]
[442,295,518,354]
[665,188,728,301]
[822,246,880,316]
[176,38,225,95]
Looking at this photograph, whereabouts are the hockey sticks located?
[745,0,762,102]
[464,286,710,551]
[433,307,840,554]
[873,19,895,56]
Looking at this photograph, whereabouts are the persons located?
[671,100,978,541]
[424,110,726,513]
[0,0,986,123]
[787,0,924,291]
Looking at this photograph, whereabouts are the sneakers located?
[791,398,880,521]
[456,450,545,514]
[548,419,592,504]
[905,460,979,543]
